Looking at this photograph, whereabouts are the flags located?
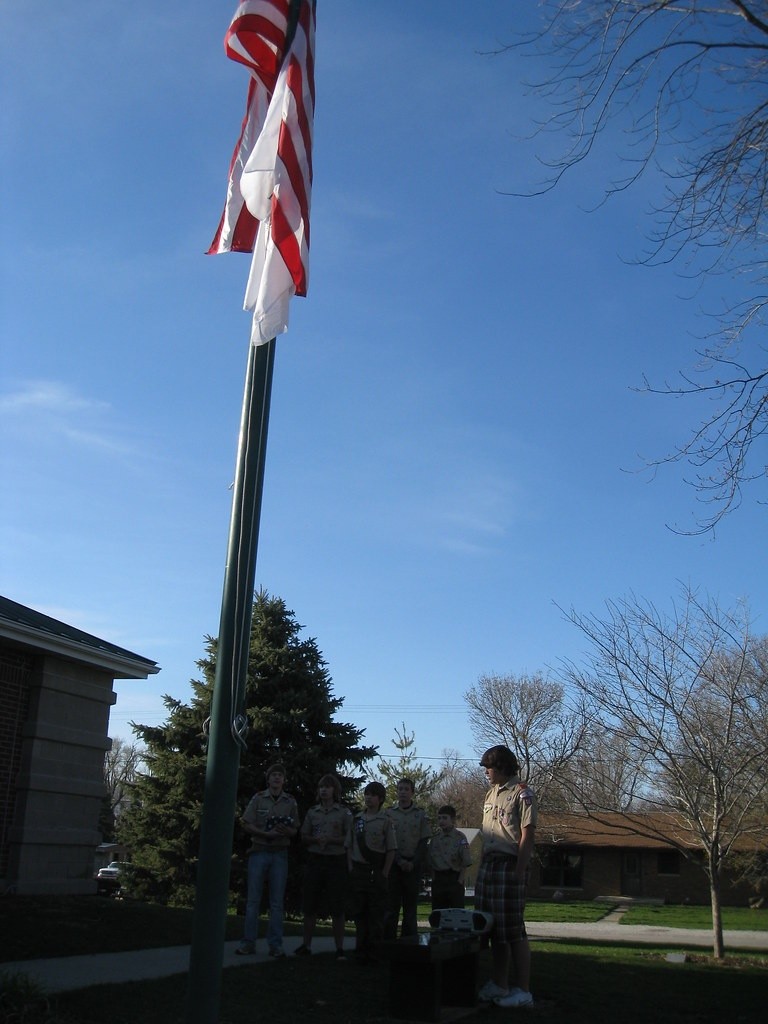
[205,0,315,346]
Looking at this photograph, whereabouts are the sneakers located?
[477,979,512,1002]
[493,987,535,1011]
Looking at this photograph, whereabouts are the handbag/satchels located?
[363,849,396,879]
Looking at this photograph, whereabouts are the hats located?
[479,745,522,771]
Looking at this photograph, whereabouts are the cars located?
[98,861,131,880]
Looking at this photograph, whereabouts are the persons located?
[232,765,300,957]
[475,746,538,1008]
[290,775,431,962]
[427,806,472,909]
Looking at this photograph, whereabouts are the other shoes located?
[334,947,347,962]
[269,944,287,958]
[294,944,313,957]
[235,940,259,955]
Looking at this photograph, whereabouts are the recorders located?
[428,908,493,932]
